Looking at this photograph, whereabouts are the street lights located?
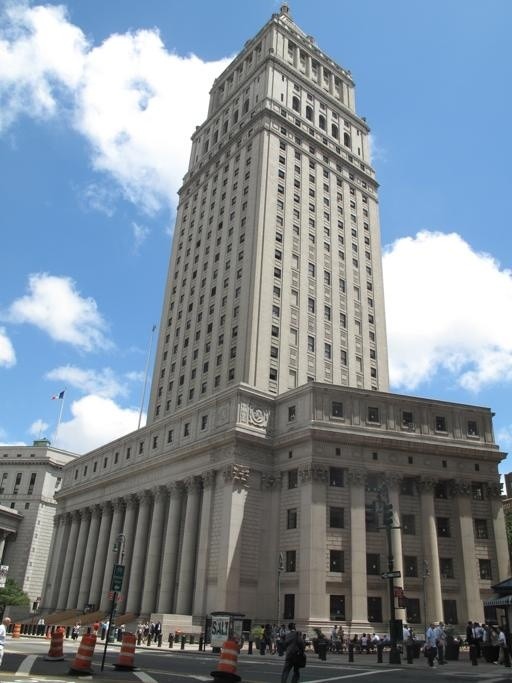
[277,552,284,627]
[422,560,429,643]
[101,535,125,671]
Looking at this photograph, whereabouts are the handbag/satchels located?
[297,654,306,667]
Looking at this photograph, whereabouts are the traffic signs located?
[111,565,125,591]
[381,571,400,579]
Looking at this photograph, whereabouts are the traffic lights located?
[383,504,393,525]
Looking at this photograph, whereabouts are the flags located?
[50,390,65,400]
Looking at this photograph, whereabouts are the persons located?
[72,620,162,645]
[242,621,512,683]
[0,617,11,667]
